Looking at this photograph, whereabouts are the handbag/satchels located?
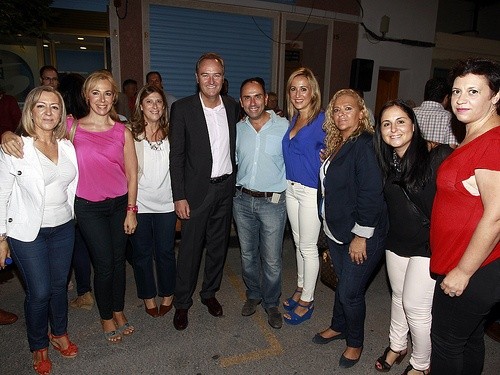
[320,249,339,292]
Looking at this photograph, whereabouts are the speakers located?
[349,59,374,91]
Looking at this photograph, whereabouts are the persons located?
[0,85,79,375]
[312,89,389,368]
[374,100,455,375]
[39,65,95,310]
[169,53,237,330]
[233,76,287,329]
[430,58,500,375]
[115,70,282,121]
[1,71,138,344]
[0,91,23,327]
[127,83,177,318]
[411,77,458,148]
[283,68,325,323]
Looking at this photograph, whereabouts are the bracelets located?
[126,206,138,212]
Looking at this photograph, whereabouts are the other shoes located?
[265,307,282,329]
[173,309,188,330]
[242,298,262,316]
[159,295,174,316]
[339,345,363,368]
[312,326,344,344]
[201,296,223,317]
[143,299,158,318]
[0,308,18,325]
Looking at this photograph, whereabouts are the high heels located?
[48,332,79,358]
[70,291,94,311]
[284,301,314,325]
[401,362,431,375]
[283,289,302,310]
[375,345,407,372]
[33,347,52,375]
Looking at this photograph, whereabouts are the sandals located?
[100,318,122,344]
[113,316,134,337]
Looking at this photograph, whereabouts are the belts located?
[236,186,286,198]
[209,172,233,184]
